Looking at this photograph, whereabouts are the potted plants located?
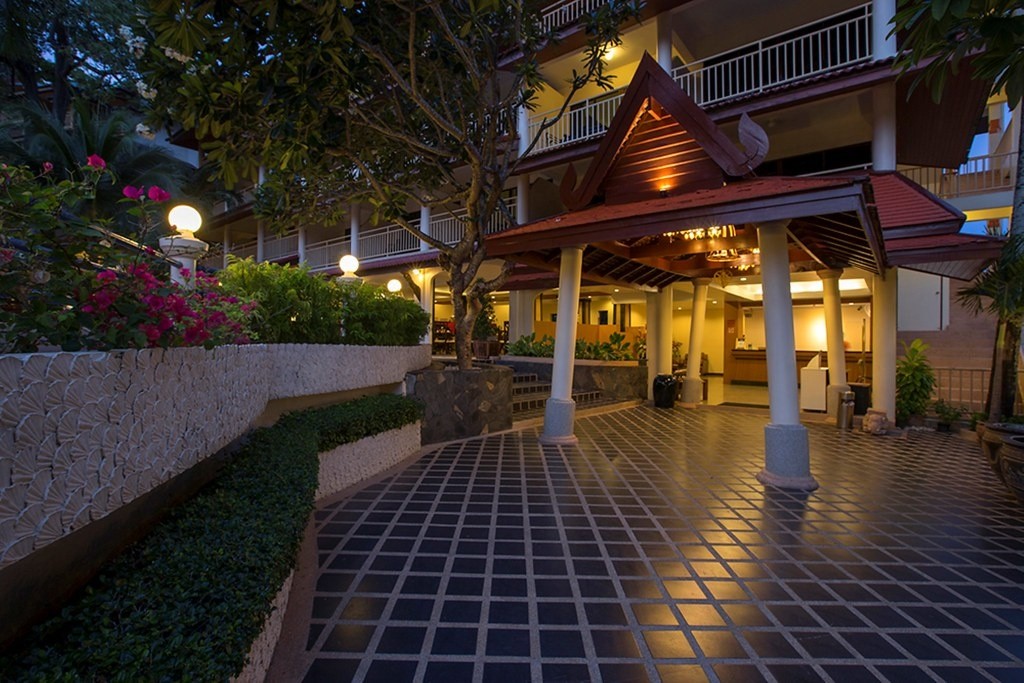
[895,337,938,426]
[472,277,500,359]
[935,398,966,432]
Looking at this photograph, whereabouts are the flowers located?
[0,24,257,348]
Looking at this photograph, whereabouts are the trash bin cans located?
[848,382,872,416]
[836,390,856,428]
[652,373,679,410]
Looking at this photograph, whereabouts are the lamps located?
[386,279,402,297]
[168,205,202,239]
[339,255,359,278]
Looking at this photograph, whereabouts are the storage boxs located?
[653,372,678,408]
[836,391,855,429]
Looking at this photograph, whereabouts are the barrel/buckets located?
[980,422,1024,492]
[997,433,1024,507]
[652,372,678,408]
[975,419,989,446]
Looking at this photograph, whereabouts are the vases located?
[1000,435,1024,501]
[976,420,994,451]
[982,423,1024,479]
